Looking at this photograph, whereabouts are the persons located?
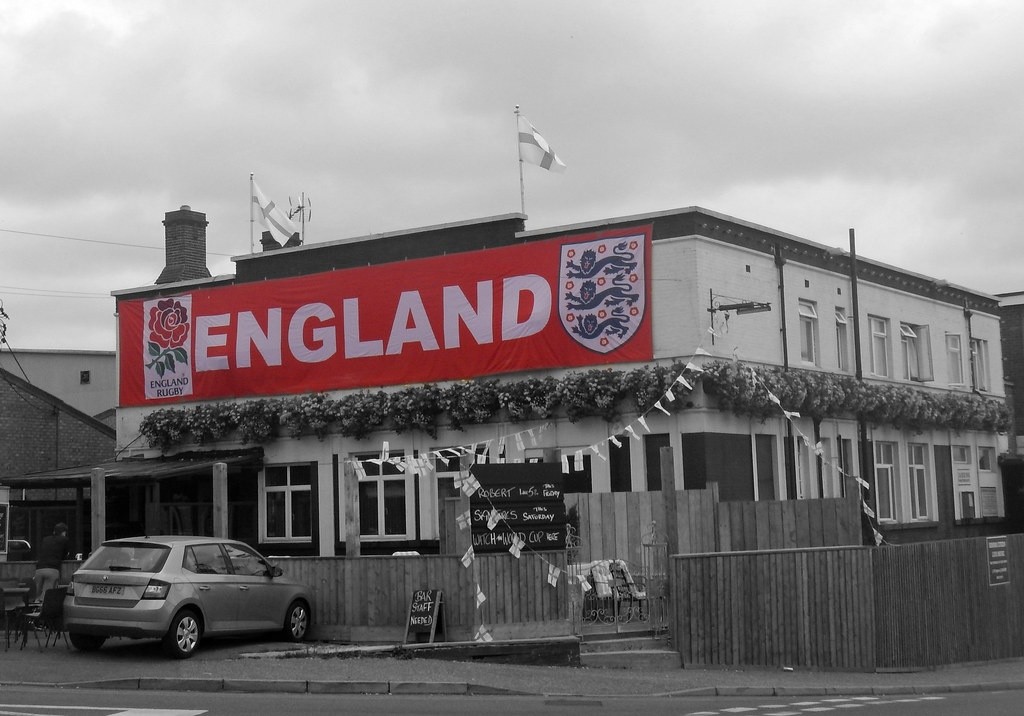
[33,523,67,605]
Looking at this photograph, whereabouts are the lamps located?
[708,301,773,316]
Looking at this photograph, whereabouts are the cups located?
[75,553,82,560]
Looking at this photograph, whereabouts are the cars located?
[60,534,315,660]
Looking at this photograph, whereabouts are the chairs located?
[0,586,68,653]
[578,559,663,622]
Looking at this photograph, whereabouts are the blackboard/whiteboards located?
[468,463,565,555]
[407,590,447,632]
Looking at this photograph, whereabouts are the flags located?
[252,181,298,247]
[518,114,567,173]
[352,327,883,643]
